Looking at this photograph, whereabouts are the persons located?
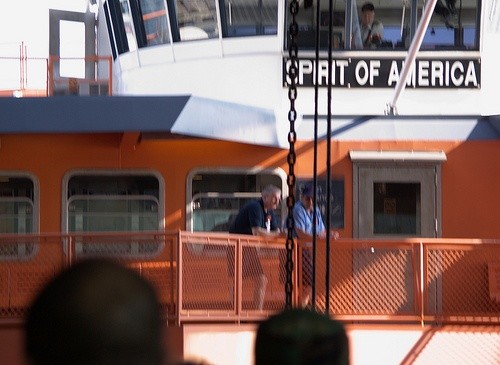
[22,256,162,365]
[358,3,383,51]
[231,184,281,312]
[251,305,350,365]
[284,187,340,311]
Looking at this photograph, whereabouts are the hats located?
[299,183,314,196]
